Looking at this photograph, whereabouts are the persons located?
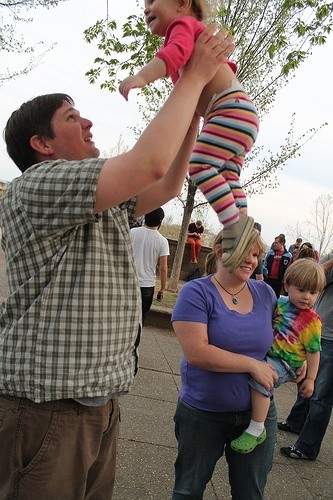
[0,21,236,499]
[229,257,327,453]
[278,248,333,461]
[117,0,261,270]
[253,223,319,300]
[187,220,204,264]
[171,227,307,500]
[130,207,170,379]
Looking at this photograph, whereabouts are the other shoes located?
[191,259,198,264]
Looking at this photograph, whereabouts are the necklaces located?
[213,275,246,305]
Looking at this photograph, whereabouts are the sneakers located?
[222,214,254,267]
[227,228,259,273]
[277,421,299,435]
[280,445,316,460]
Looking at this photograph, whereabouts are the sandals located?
[230,426,266,454]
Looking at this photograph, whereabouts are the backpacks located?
[186,268,201,281]
